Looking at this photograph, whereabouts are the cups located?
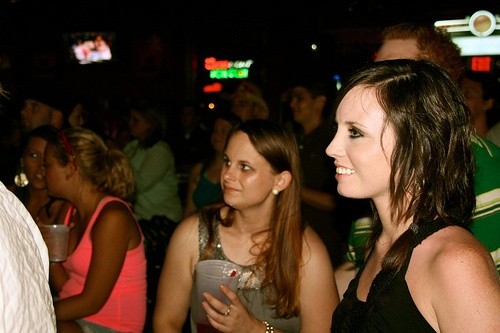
[196,260,241,324]
[44,225,69,262]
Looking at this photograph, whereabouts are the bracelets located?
[263,320,274,333]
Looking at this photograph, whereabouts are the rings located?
[225,308,231,316]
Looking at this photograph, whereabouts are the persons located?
[0,23,500,333]
